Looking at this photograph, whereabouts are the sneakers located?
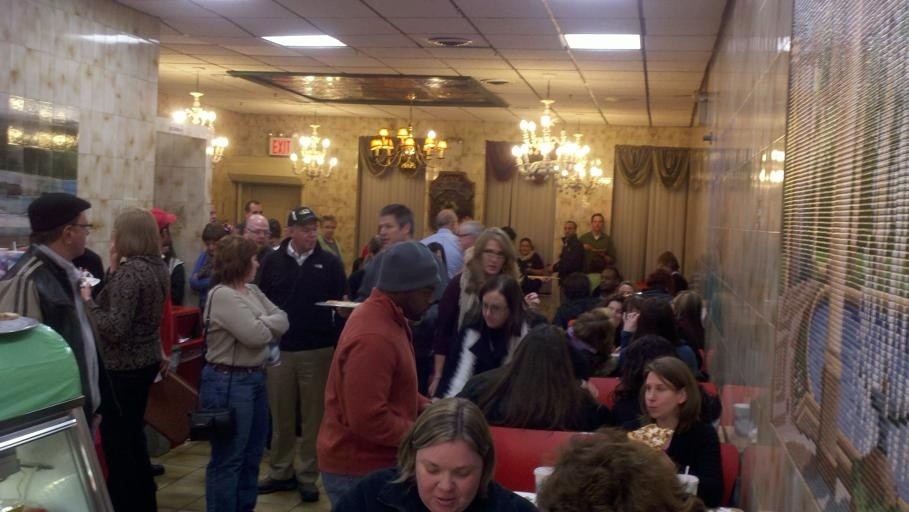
[298,479,322,505]
[255,475,296,504]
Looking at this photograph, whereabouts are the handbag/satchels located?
[192,284,240,442]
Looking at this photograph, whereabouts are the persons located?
[190,201,724,511]
[1,192,186,511]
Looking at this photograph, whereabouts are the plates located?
[83,278,102,288]
[314,300,363,308]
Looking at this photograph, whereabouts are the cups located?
[734,401,751,438]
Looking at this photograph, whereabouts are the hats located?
[287,206,320,226]
[377,241,442,293]
[27,191,93,232]
[148,208,177,232]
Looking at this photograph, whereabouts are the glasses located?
[245,226,273,236]
[482,247,506,258]
[71,222,93,230]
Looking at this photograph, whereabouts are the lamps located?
[170,65,217,130]
[511,97,571,186]
[203,136,230,165]
[357,92,449,180]
[266,130,299,138]
[552,129,614,209]
[288,108,339,188]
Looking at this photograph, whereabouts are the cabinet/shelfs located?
[0,309,118,512]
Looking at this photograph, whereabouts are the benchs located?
[487,315,743,510]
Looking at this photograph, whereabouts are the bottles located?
[532,466,555,496]
[677,473,699,496]
